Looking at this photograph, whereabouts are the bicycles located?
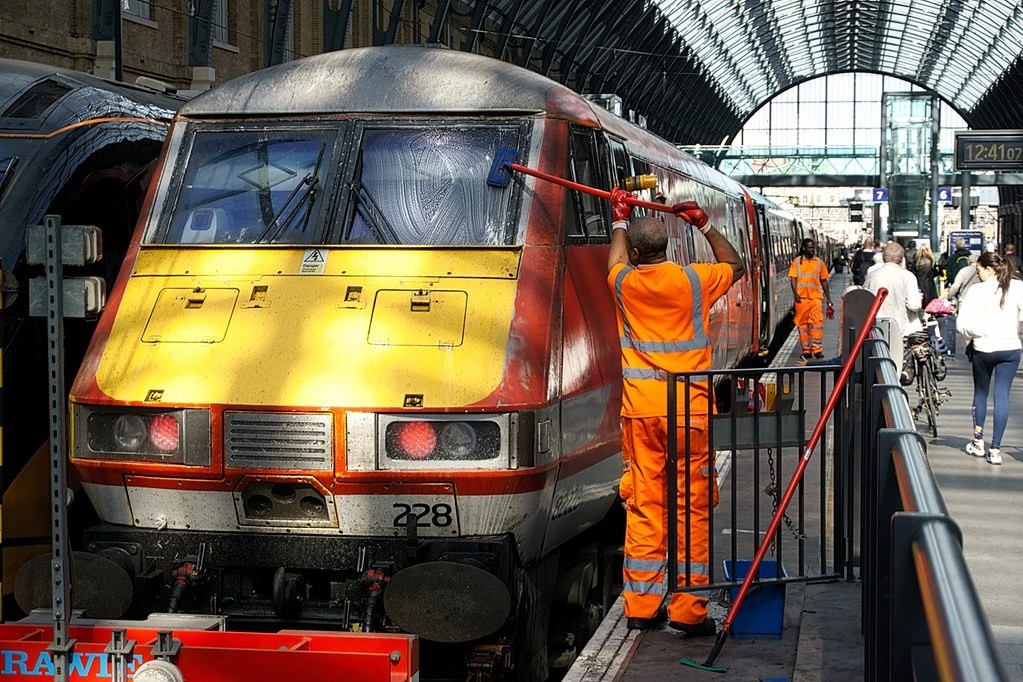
[901,333,948,438]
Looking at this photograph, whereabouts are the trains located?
[68,45,845,682]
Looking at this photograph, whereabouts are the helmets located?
[907,331,930,346]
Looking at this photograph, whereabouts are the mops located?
[678,286,888,674]
[487,148,707,220]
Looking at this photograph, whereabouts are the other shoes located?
[627,608,667,631]
[800,353,811,359]
[669,617,716,637]
[986,448,1002,464]
[966,439,985,457]
[815,353,824,358]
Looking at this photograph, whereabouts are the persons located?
[958,252,1023,464]
[788,239,834,359]
[608,187,746,636]
[832,239,1023,380]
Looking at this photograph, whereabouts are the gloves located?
[672,201,710,230]
[609,187,638,222]
[825,307,836,321]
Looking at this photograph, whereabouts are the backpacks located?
[951,257,970,280]
[856,253,875,285]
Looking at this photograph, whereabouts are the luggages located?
[928,298,956,353]
